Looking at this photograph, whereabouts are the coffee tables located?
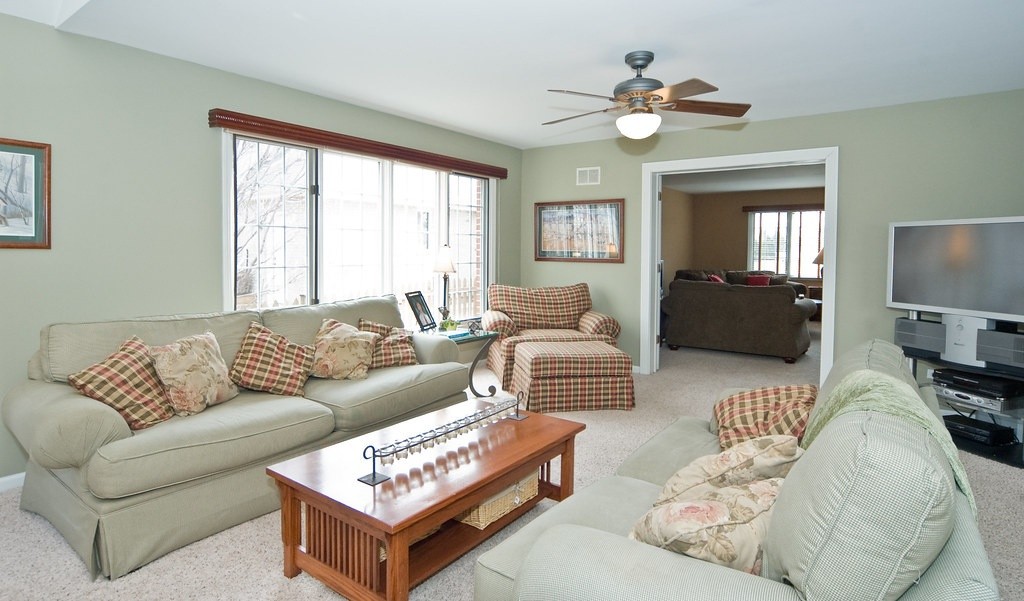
[266,399,587,601]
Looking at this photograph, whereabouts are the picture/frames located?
[533,199,625,264]
[405,291,437,331]
[0,137,52,250]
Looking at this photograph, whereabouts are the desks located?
[795,297,822,304]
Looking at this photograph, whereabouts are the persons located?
[414,299,431,325]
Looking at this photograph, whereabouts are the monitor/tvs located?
[885,216,1024,324]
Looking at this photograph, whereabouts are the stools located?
[510,341,635,413]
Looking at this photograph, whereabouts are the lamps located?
[813,248,823,278]
[616,98,662,140]
[433,244,456,321]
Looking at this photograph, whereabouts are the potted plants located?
[439,318,460,331]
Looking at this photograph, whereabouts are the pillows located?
[359,318,419,368]
[68,335,174,430]
[708,271,788,286]
[228,321,316,396]
[148,331,239,417]
[629,478,785,576]
[715,383,818,453]
[312,319,381,380]
[653,435,805,507]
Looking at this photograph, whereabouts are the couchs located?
[473,340,1001,601]
[661,279,818,364]
[0,293,468,582]
[481,282,620,388]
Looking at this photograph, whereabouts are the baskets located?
[453,471,539,531]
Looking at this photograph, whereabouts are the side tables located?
[432,327,499,397]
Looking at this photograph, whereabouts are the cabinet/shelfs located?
[901,345,1024,470]
[808,286,822,322]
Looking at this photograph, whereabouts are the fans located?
[542,51,752,125]
